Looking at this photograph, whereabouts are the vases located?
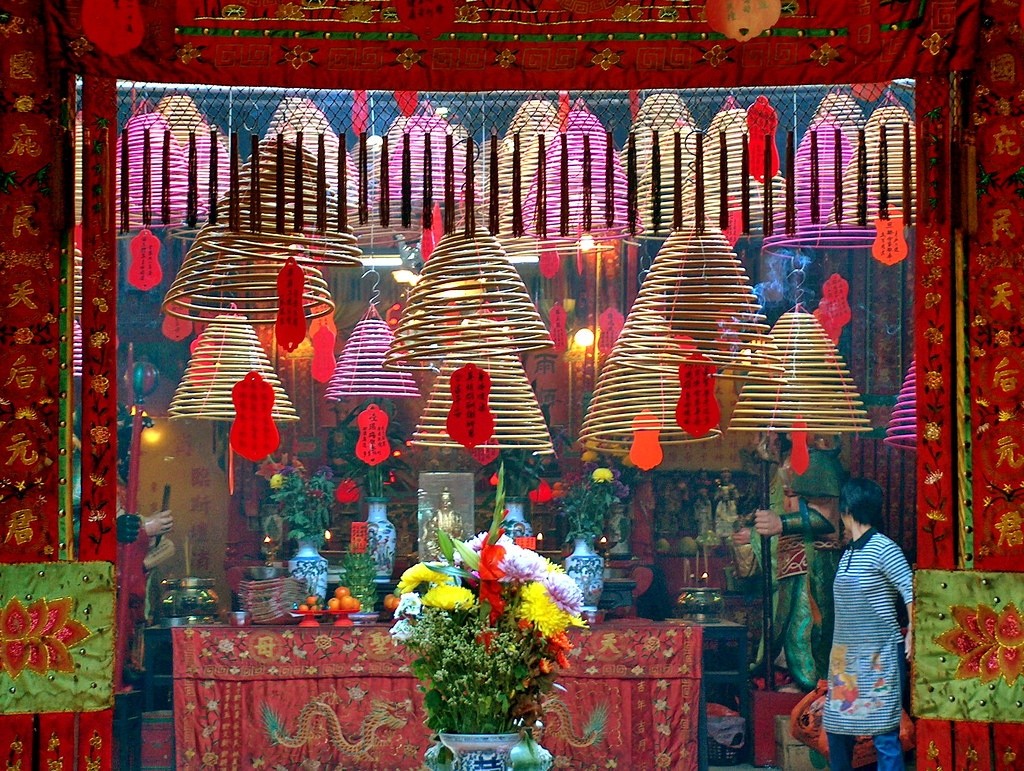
[500,495,533,544]
[287,536,329,601]
[439,728,519,771]
[607,505,632,553]
[563,534,604,614]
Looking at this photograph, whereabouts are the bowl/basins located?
[247,566,282,580]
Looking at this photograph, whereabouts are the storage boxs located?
[774,714,829,771]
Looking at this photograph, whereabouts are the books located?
[143,533,176,569]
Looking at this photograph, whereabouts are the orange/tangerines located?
[383,594,401,611]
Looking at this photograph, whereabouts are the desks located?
[172,617,702,771]
[664,618,752,771]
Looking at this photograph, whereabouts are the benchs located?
[142,624,174,712]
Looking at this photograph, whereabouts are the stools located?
[113,690,143,771]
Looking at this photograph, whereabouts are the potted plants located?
[331,396,412,583]
[335,540,380,625]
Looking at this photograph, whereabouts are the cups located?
[234,612,246,626]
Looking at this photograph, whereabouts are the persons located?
[823,476,913,771]
[663,426,842,691]
[114,399,173,673]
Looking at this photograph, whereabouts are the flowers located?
[582,435,646,503]
[255,453,336,552]
[388,462,592,771]
[473,378,571,510]
[552,459,629,552]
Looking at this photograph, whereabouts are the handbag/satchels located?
[790,678,916,769]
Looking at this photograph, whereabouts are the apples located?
[297,586,360,610]
